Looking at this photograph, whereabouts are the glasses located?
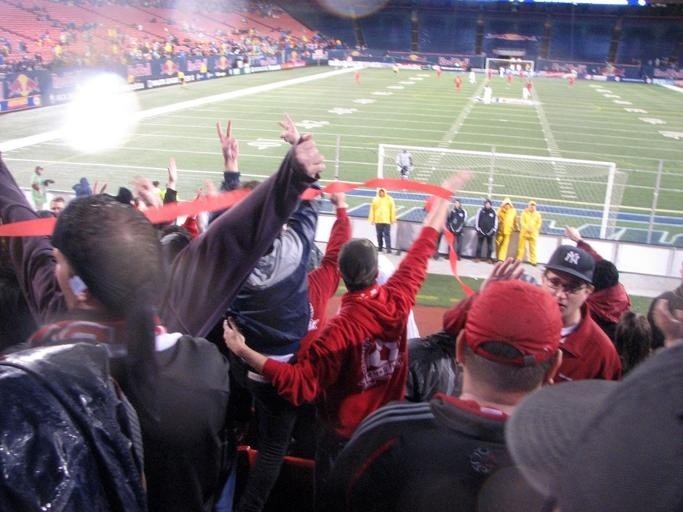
[544,273,589,295]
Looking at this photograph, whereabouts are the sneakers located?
[485,258,494,265]
[445,255,449,259]
[456,256,460,261]
[474,256,481,263]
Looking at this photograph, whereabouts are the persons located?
[0,110,683,511]
[354,70,360,85]
[0,111,683,512]
[434,64,441,79]
[454,75,461,90]
[1,0,343,113]
[565,67,578,89]
[469,66,534,105]
[391,64,400,81]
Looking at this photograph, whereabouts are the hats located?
[528,201,537,206]
[463,280,564,367]
[546,245,596,283]
